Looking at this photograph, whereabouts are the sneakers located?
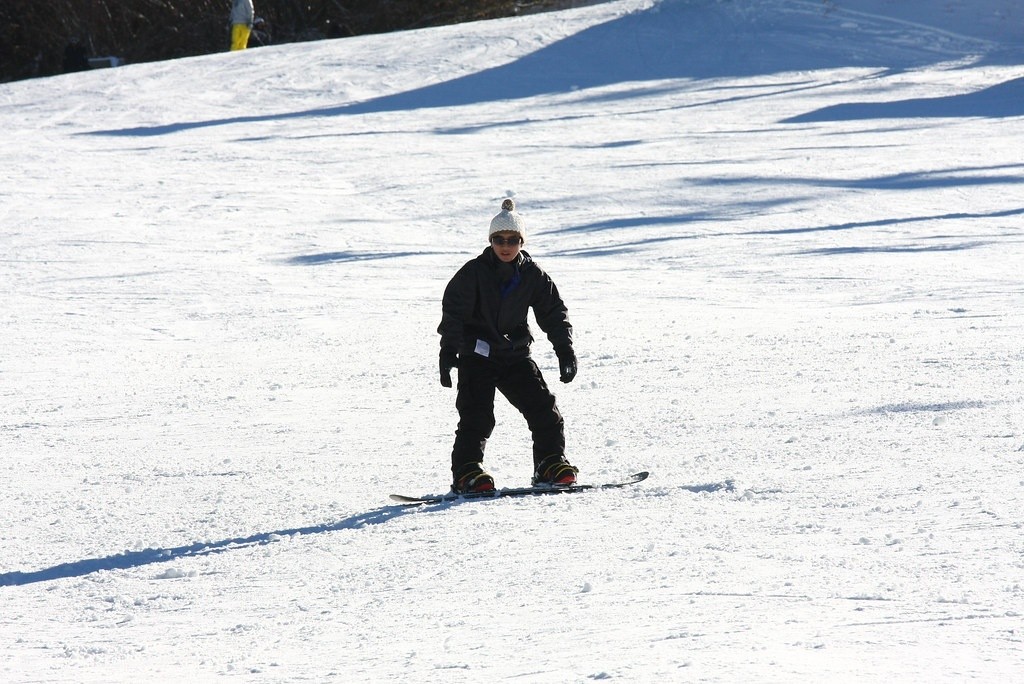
[529,452,579,489]
[450,458,496,495]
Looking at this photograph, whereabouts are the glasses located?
[490,235,523,246]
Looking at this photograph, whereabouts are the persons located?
[228,0,255,51]
[436,198,579,494]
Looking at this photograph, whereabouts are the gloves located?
[557,352,578,384]
[437,351,460,389]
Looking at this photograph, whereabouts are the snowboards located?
[389,469,651,505]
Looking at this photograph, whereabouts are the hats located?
[488,198,527,241]
